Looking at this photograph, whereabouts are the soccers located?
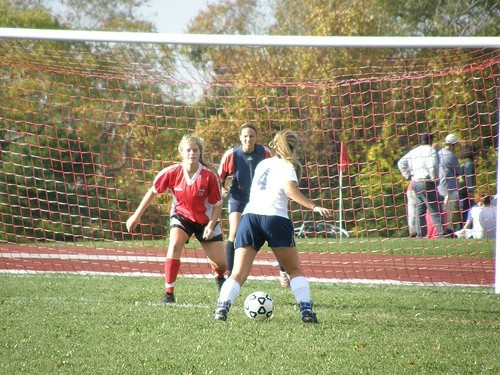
[243,291,275,321]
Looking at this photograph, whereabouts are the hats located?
[445,134,459,144]
[421,133,437,141]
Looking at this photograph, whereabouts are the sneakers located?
[212,300,232,321]
[160,291,176,303]
[297,300,317,323]
[280,270,290,288]
[215,276,226,292]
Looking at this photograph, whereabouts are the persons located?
[397,131,497,239]
[211,123,331,324]
[125,134,227,304]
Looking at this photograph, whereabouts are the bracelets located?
[310,204,320,210]
